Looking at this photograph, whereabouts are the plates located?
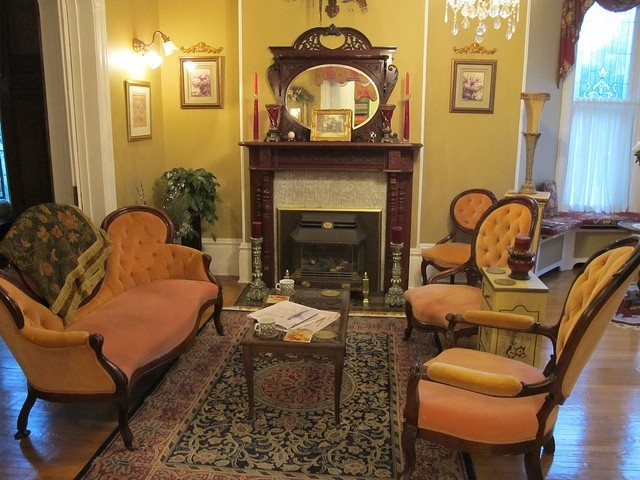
[255,331,279,339]
[278,291,295,296]
[314,329,338,341]
[320,289,343,298]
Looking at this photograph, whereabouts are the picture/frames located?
[178,55,223,110]
[124,79,153,143]
[310,108,352,142]
[449,59,497,114]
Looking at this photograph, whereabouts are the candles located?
[253,71,258,99]
[392,224,403,243]
[251,220,263,238]
[405,72,410,97]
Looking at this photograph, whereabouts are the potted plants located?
[160,166,221,252]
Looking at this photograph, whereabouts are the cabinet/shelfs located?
[476,266,550,372]
[236,140,423,296]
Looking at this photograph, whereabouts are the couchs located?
[400,192,539,349]
[395,233,640,479]
[420,186,498,289]
[1,201,225,452]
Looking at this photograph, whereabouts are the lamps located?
[442,0,522,46]
[132,30,180,71]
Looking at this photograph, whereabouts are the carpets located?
[72,306,478,479]
[611,280,640,327]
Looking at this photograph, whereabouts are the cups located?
[275,278,295,293]
[253,317,276,336]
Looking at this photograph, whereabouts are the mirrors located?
[284,63,382,133]
